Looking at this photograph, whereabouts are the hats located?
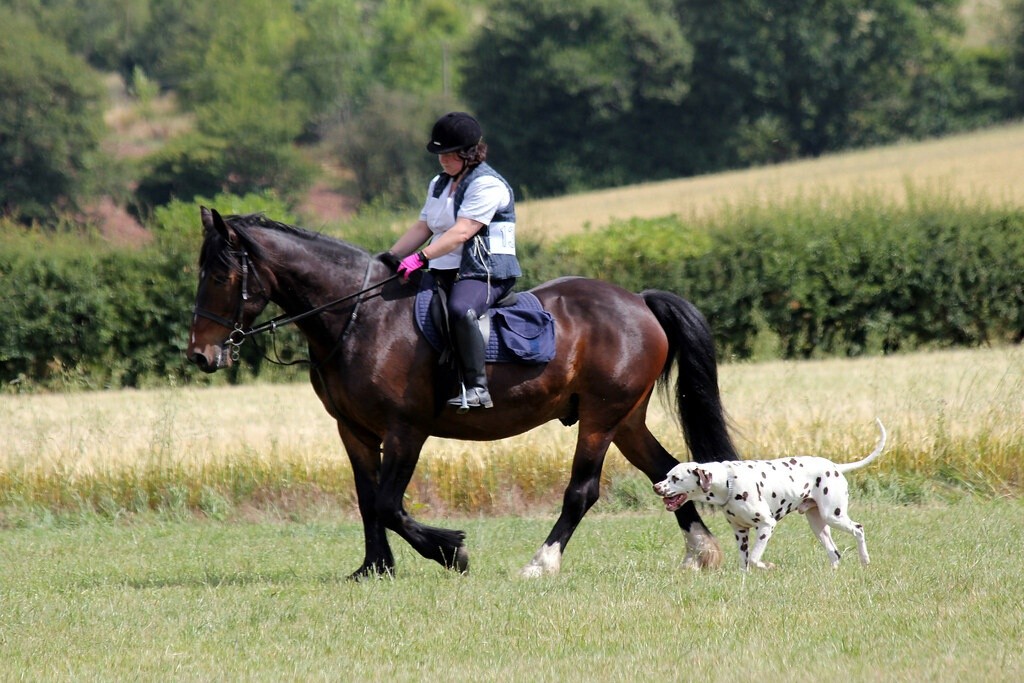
[427,110,482,154]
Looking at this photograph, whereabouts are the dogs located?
[652,416,887,570]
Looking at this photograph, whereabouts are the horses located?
[185,204,743,582]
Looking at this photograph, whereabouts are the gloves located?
[398,249,429,281]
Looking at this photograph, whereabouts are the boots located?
[447,309,494,410]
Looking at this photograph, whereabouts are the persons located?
[385,112,523,413]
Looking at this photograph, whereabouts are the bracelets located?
[418,250,431,262]
